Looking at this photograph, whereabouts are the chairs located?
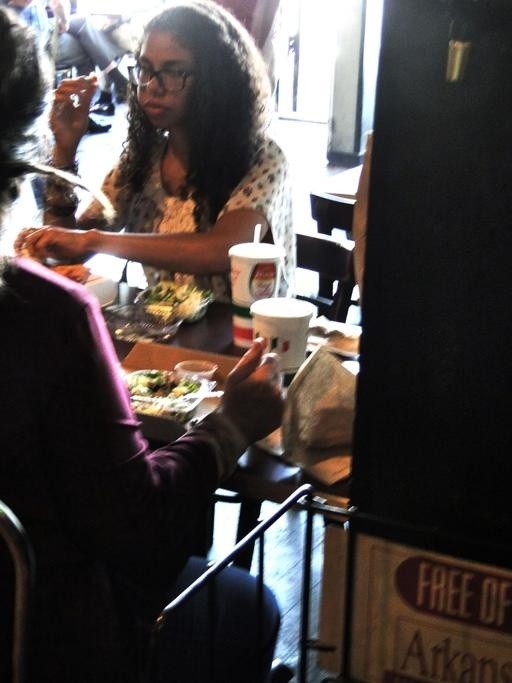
[214,230,360,584]
[310,192,359,327]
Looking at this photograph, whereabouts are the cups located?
[248,294,321,375]
[228,242,288,351]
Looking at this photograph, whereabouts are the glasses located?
[135,64,197,92]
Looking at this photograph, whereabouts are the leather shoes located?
[89,103,114,115]
[86,116,112,134]
[114,77,129,104]
